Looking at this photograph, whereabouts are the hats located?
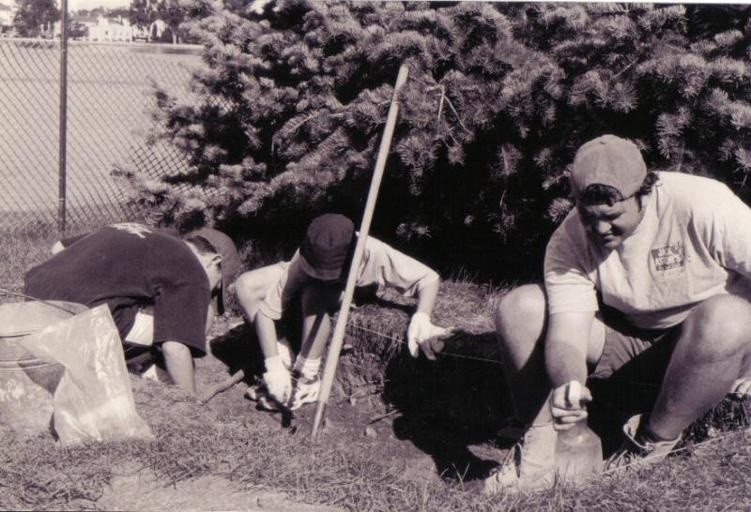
[299,214,355,281]
[570,134,648,204]
[191,227,240,314]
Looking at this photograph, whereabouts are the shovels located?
[279,392,297,419]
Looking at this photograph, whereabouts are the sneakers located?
[247,363,321,410]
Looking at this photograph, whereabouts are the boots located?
[481,424,558,496]
[603,414,682,477]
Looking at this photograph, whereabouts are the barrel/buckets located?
[1,286,91,434]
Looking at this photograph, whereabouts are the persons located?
[19,220,242,397]
[458,131,750,479]
[232,211,457,413]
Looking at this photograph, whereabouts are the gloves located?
[263,356,292,407]
[407,313,455,361]
